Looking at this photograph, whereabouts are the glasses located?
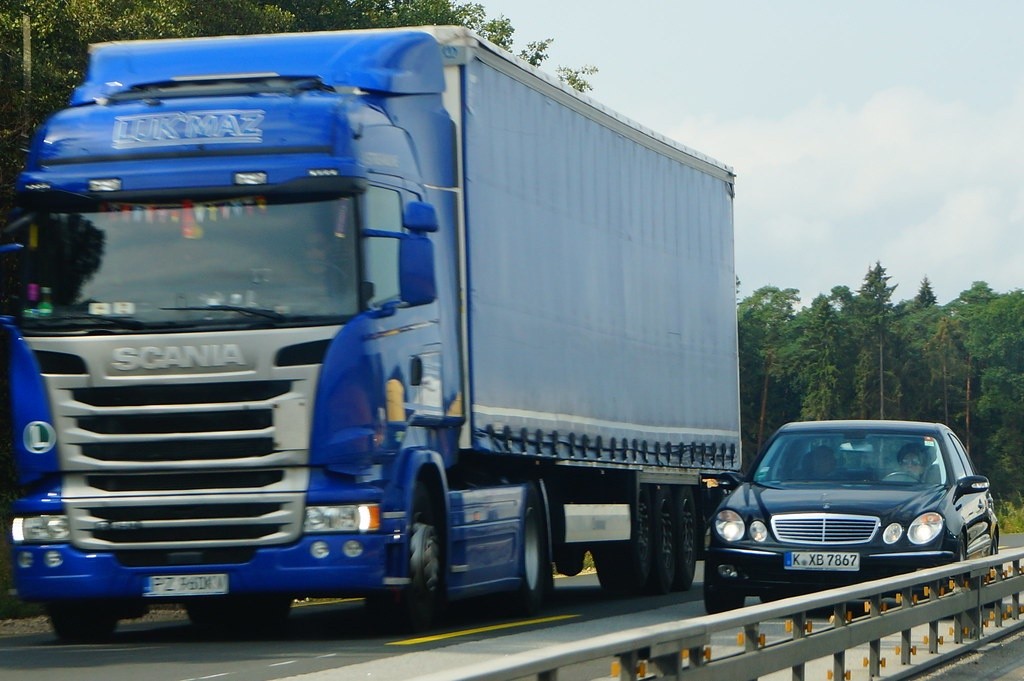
[902,458,923,466]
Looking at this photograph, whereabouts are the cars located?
[704,419,1003,617]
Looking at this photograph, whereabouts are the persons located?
[888,446,926,482]
[800,446,845,481]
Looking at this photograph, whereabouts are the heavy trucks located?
[1,23,745,647]
